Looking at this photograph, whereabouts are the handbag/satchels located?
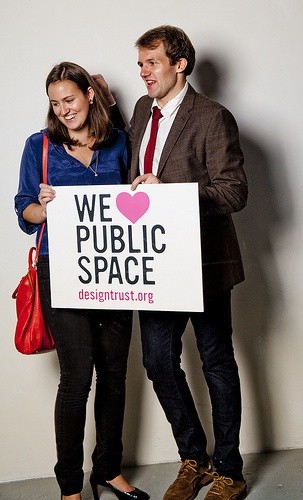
[12,247,56,355]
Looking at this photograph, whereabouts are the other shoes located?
[204,471,248,500]
[163,460,214,500]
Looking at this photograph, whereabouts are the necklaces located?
[78,147,99,177]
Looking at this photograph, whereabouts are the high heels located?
[90,474,150,500]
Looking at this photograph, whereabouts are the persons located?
[15,62,151,500]
[92,25,249,500]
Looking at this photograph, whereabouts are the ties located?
[144,106,162,174]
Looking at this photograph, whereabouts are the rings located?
[38,195,41,199]
